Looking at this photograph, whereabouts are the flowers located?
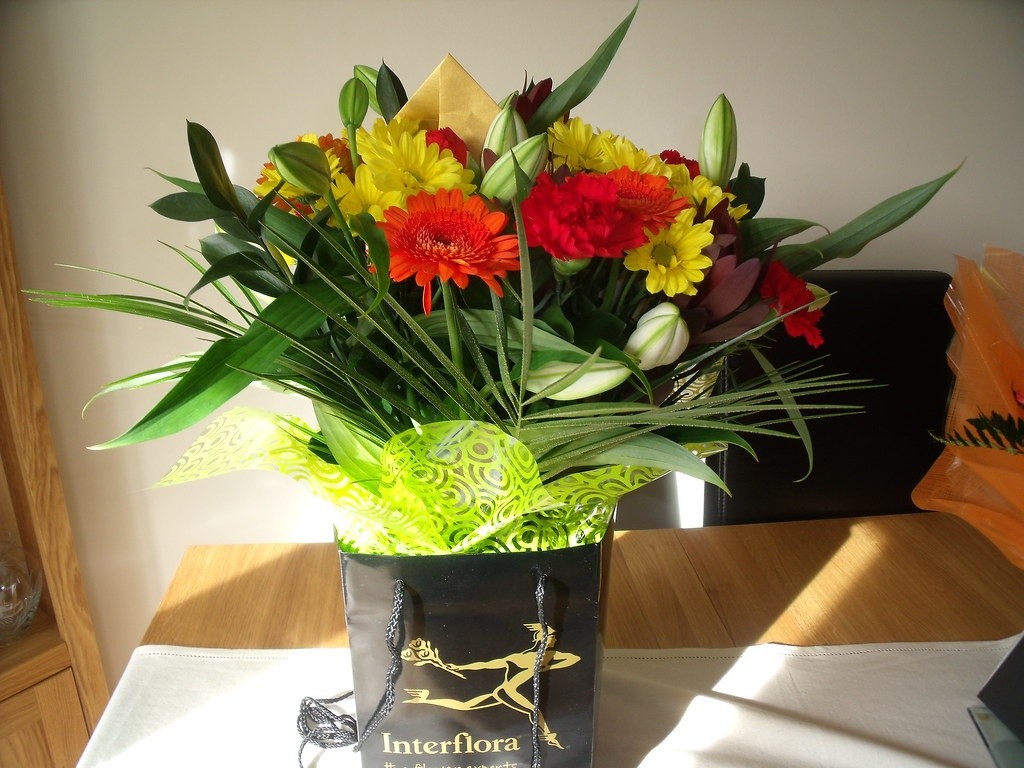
[23,0,969,555]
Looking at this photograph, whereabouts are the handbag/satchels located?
[297,503,618,768]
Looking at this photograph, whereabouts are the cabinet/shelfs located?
[0,163,112,768]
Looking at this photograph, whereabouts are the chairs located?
[703,270,958,526]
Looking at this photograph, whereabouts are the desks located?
[75,511,1023,768]
